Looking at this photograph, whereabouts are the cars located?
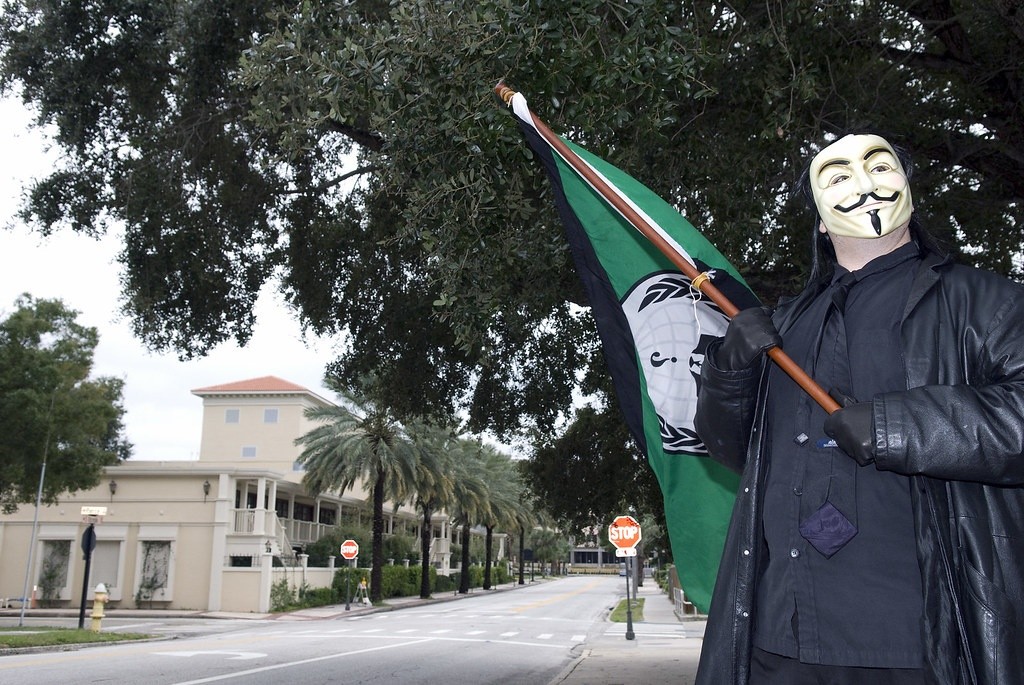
[619,569,626,576]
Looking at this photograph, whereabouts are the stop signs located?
[340,540,359,560]
[609,516,643,549]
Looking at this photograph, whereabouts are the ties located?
[798,272,858,520]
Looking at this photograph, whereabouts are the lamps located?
[204,480,211,495]
[109,479,117,494]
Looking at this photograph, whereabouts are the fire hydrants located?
[87,582,110,633]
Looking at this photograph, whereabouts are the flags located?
[512,96,755,613]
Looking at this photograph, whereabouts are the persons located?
[692,134,1024,683]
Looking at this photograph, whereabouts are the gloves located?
[713,305,781,371]
[823,387,877,467]
